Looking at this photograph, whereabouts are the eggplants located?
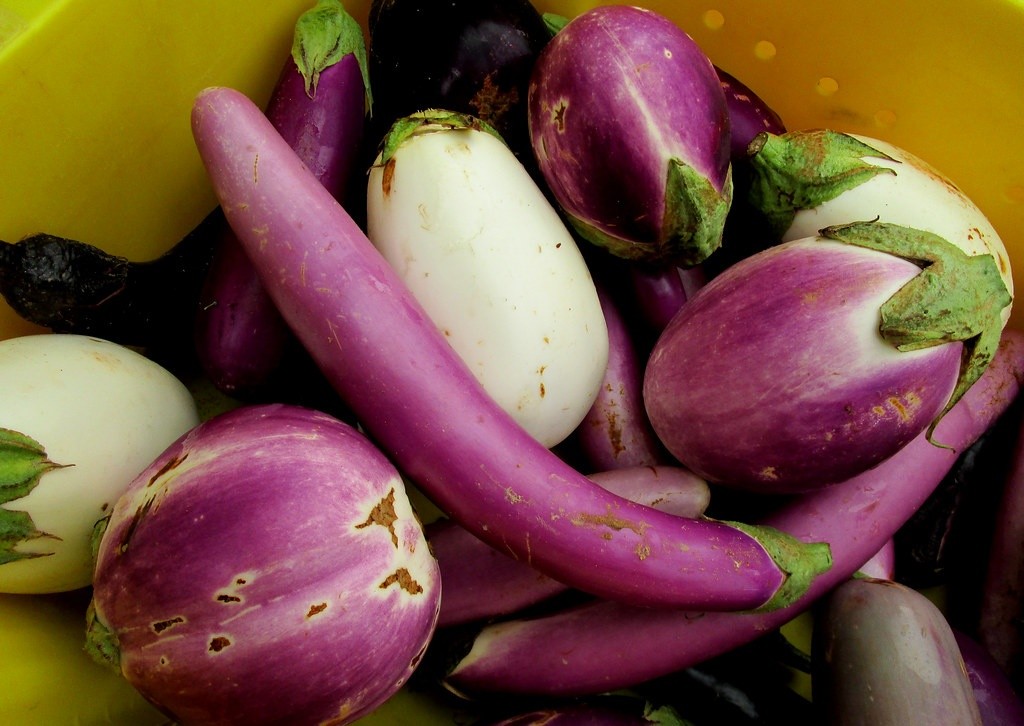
[2,0,1023,726]
